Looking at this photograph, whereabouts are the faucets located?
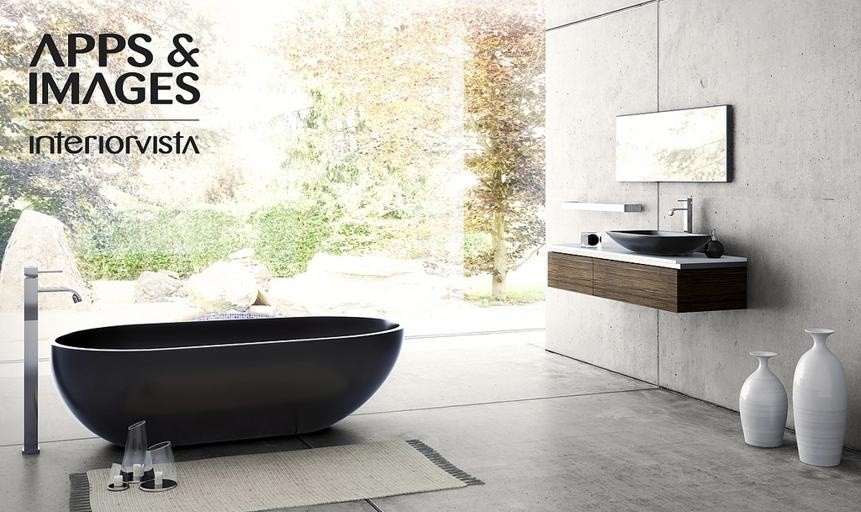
[668,196,693,234]
[36,285,84,307]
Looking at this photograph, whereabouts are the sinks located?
[604,228,713,257]
[49,315,405,446]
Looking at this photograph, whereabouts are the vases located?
[739,351,788,448]
[792,327,849,467]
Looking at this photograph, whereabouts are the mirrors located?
[615,105,732,183]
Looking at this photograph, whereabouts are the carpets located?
[69,437,485,512]
